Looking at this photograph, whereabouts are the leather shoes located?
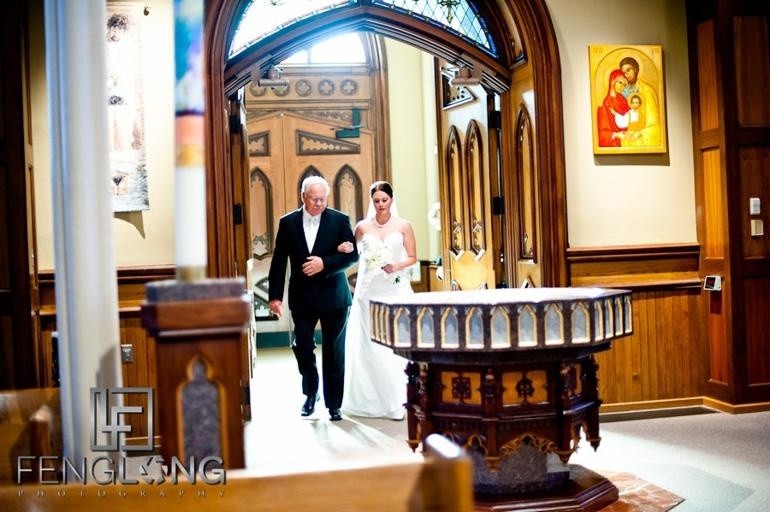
[327,408,342,419]
[299,390,320,415]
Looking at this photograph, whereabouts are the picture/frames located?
[588,43,668,155]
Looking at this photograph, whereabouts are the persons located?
[596,55,663,149]
[332,180,418,421]
[266,174,359,422]
[106,12,143,157]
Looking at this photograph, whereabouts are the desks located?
[370,286,634,512]
